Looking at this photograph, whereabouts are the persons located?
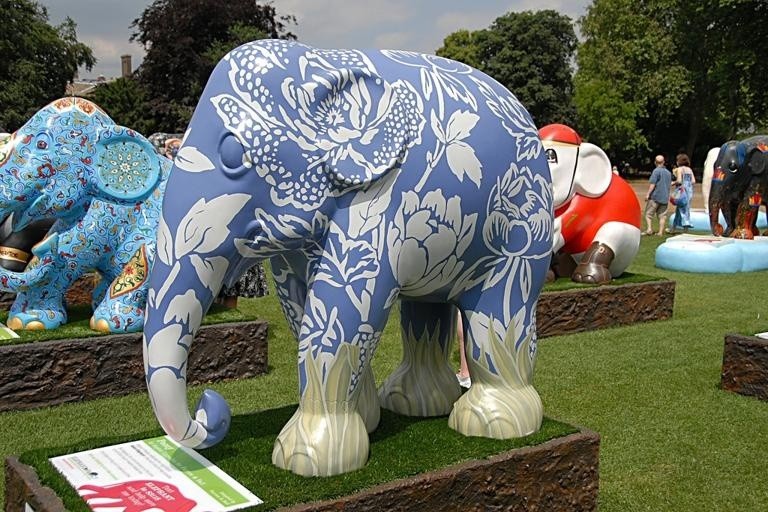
[612,162,631,181]
[664,153,696,234]
[641,155,672,238]
[665,160,678,182]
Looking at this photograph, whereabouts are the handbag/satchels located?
[670,187,687,206]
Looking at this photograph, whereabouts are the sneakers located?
[641,229,677,235]
